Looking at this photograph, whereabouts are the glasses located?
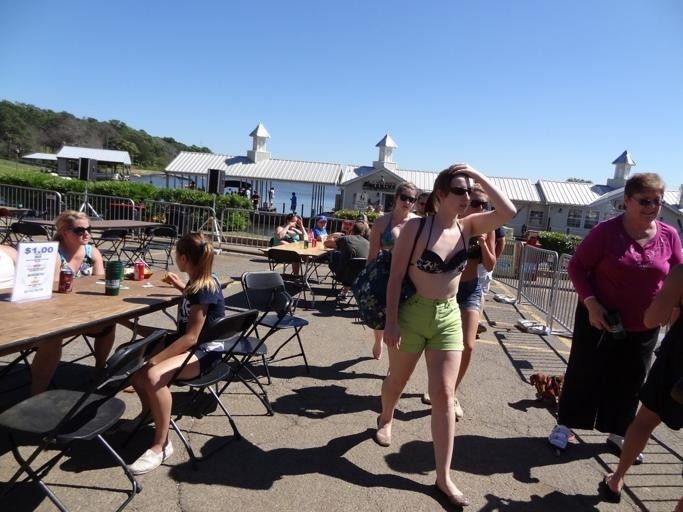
[400,193,417,203]
[630,194,666,206]
[471,199,488,210]
[73,226,92,235]
[450,187,472,195]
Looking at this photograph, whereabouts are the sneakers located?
[607,434,643,465]
[130,441,173,475]
[549,425,571,449]
[121,410,154,432]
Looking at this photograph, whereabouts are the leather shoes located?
[376,415,392,446]
[373,343,381,360]
[435,479,470,507]
[424,391,463,418]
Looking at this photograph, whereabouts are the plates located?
[124,270,151,279]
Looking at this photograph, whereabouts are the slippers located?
[603,472,623,502]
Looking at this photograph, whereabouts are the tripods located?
[193,195,231,249]
[78,183,105,220]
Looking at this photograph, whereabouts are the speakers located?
[207,168,225,194]
[78,157,96,181]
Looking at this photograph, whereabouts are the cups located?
[105,261,123,296]
[303,240,308,249]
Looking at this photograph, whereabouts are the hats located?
[316,215,327,223]
[357,215,367,222]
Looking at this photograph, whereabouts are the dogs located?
[530,372,565,399]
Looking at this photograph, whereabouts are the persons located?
[422,181,507,421]
[365,198,384,213]
[0,244,24,298]
[476,220,506,342]
[597,262,683,504]
[323,220,369,305]
[40,168,59,175]
[367,180,423,361]
[546,171,683,466]
[127,226,226,476]
[225,186,298,214]
[28,208,137,397]
[312,214,344,242]
[373,161,519,509]
[269,212,309,280]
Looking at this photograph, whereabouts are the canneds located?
[133,262,145,281]
[303,240,308,249]
[59,269,73,293]
[312,239,316,248]
